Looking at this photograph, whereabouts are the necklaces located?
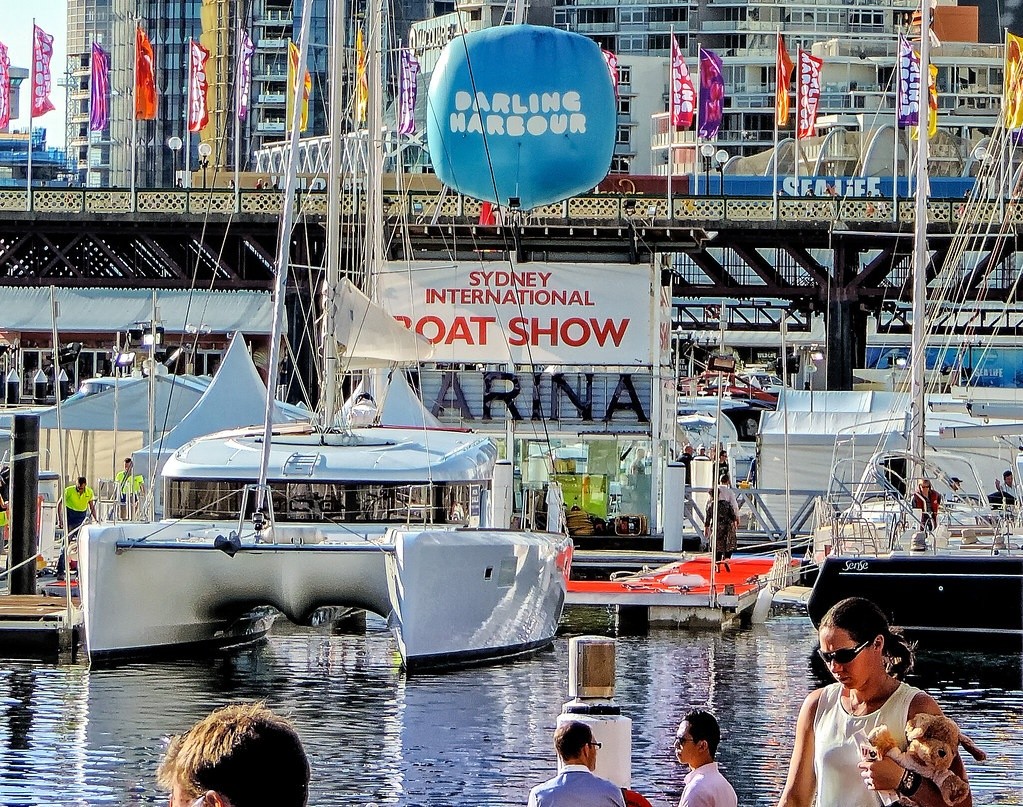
[849,675,889,715]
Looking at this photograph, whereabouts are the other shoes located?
[724,560,731,572]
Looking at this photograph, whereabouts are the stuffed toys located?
[868,713,988,807]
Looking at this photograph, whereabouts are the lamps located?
[251,507,269,530]
[623,199,636,216]
[691,342,736,373]
[809,349,825,362]
[115,321,164,367]
[894,352,909,366]
[383,197,392,213]
[508,198,520,214]
[413,202,423,214]
[647,205,658,216]
[45,341,83,368]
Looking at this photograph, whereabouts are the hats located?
[949,477,963,483]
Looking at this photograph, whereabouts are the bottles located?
[571,503,579,511]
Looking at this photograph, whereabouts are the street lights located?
[983,154,993,203]
[167,136,183,188]
[974,146,986,202]
[701,143,715,196]
[198,143,211,189]
[715,150,728,196]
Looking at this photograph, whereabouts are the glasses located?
[159,733,205,796]
[817,637,880,664]
[584,742,601,750]
[921,485,929,489]
[675,737,705,746]
[722,455,727,458]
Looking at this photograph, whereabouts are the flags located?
[0,22,1023,145]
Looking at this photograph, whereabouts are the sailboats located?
[0,0,1023,680]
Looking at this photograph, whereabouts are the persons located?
[995,470,1021,499]
[632,441,756,574]
[946,477,963,502]
[528,722,626,807]
[57,475,98,580]
[912,480,941,532]
[0,476,9,554]
[621,709,738,807]
[115,458,147,501]
[777,596,972,807]
